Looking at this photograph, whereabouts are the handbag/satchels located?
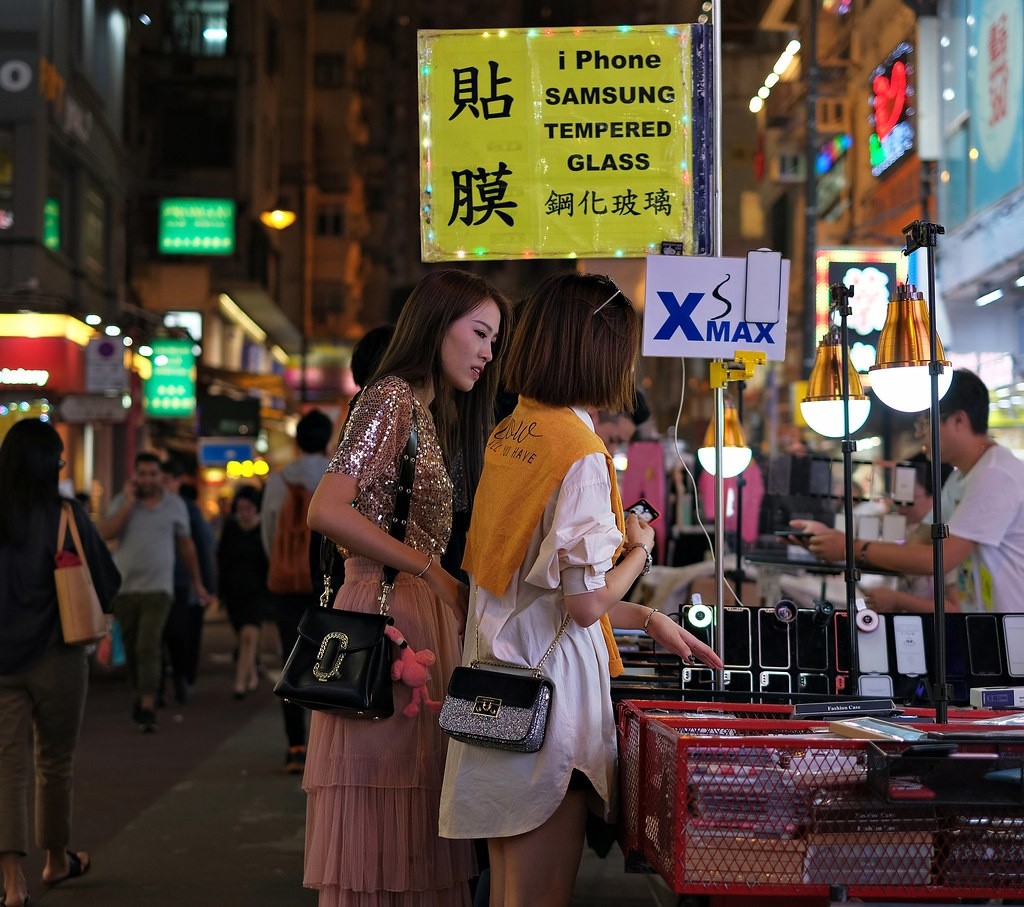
[271,606,392,726]
[49,496,113,645]
[437,655,554,754]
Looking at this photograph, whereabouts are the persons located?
[0,418,123,907]
[774,370,1024,615]
[261,407,334,774]
[100,452,211,734]
[625,505,653,522]
[438,273,723,907]
[351,325,396,389]
[588,386,651,458]
[301,271,513,907]
[865,454,961,613]
[154,482,281,710]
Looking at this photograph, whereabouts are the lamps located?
[697,388,752,479]
[867,246,952,413]
[799,302,871,438]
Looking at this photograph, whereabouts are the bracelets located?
[862,542,870,561]
[415,557,432,578]
[645,608,658,634]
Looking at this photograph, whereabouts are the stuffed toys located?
[385,625,442,717]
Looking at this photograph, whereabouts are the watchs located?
[627,543,652,576]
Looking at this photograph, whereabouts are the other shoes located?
[282,747,308,774]
[231,687,246,700]
[130,699,158,728]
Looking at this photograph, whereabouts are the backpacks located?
[265,469,334,595]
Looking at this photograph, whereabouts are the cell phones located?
[775,530,815,538]
[623,498,659,525]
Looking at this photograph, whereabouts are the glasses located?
[893,499,917,508]
[593,279,629,316]
[909,412,951,428]
[53,456,67,472]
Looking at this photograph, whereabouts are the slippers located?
[38,849,94,887]
